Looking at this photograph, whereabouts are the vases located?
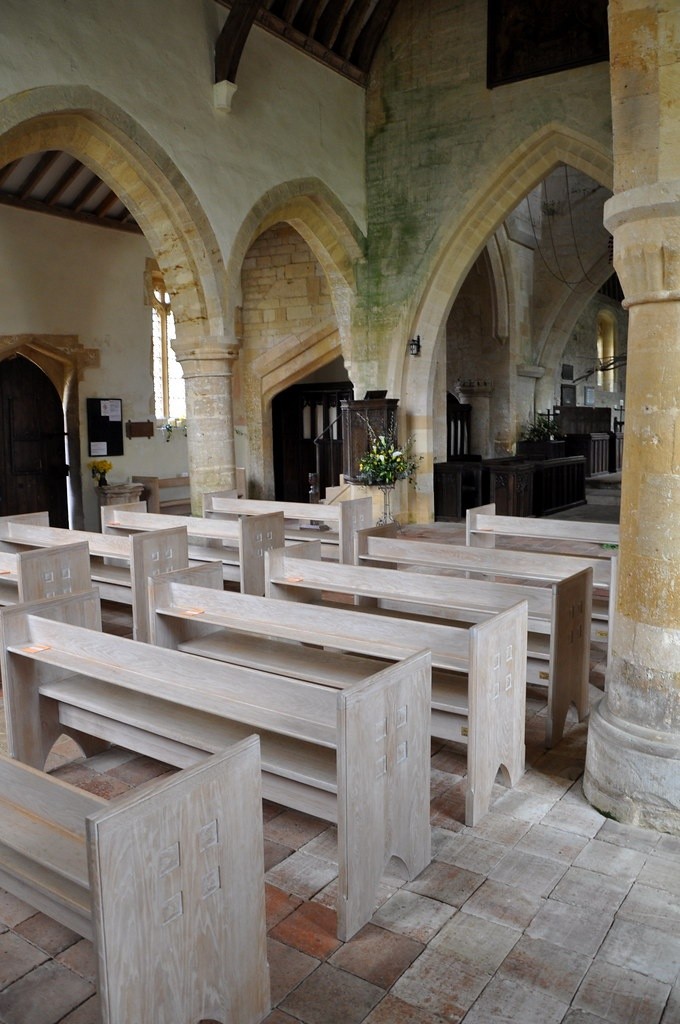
[98,471,107,487]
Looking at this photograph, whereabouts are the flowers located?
[346,409,425,487]
[88,460,113,480]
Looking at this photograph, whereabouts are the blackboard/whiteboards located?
[86,397,124,457]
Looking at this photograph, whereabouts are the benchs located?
[0,469,621,1024]
